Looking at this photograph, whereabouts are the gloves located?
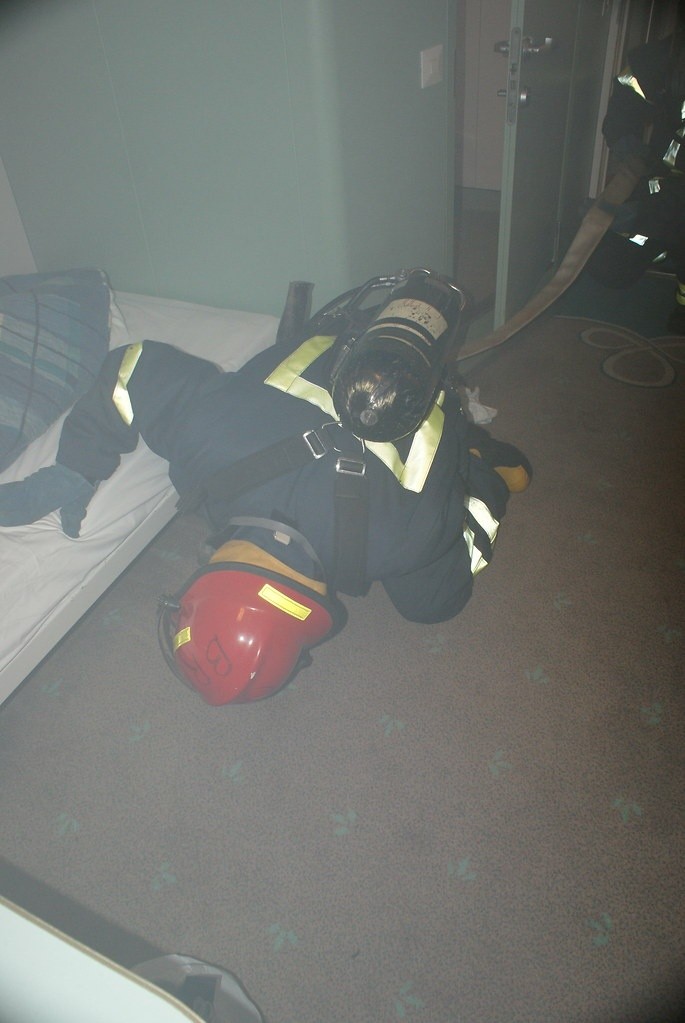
[599,199,640,235]
[612,135,657,181]
[0,459,99,539]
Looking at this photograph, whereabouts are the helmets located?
[158,563,340,705]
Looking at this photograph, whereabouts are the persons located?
[0,308,531,707]
[602,35,685,336]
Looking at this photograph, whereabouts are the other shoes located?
[667,305,685,336]
[464,425,533,494]
[605,256,650,290]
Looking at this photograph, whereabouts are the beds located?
[0,290,284,700]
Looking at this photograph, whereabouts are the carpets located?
[543,274,685,338]
